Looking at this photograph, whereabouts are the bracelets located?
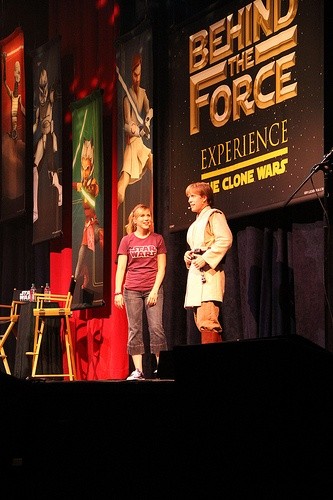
[115,292,122,295]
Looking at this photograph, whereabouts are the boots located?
[201,330,222,344]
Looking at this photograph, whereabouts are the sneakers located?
[126,370,144,380]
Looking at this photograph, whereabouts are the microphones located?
[314,147,333,172]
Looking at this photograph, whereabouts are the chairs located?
[25,292,73,381]
[0,301,28,375]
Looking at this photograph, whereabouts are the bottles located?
[44,283,50,302]
[30,284,36,302]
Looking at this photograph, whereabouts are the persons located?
[114,204,167,380]
[183,183,233,344]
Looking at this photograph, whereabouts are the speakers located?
[167,333,333,382]
